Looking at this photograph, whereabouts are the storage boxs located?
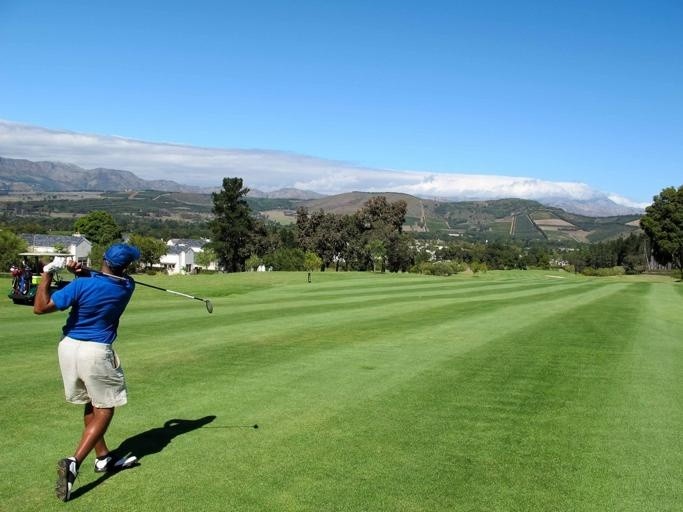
[31,276,42,285]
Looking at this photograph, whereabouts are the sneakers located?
[56,456,78,502]
[94,453,138,472]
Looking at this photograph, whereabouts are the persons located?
[31,244,143,503]
[12,255,47,280]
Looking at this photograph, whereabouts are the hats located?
[105,244,141,269]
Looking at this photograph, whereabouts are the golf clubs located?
[80,265,212,313]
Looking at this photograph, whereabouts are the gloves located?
[42,256,66,273]
